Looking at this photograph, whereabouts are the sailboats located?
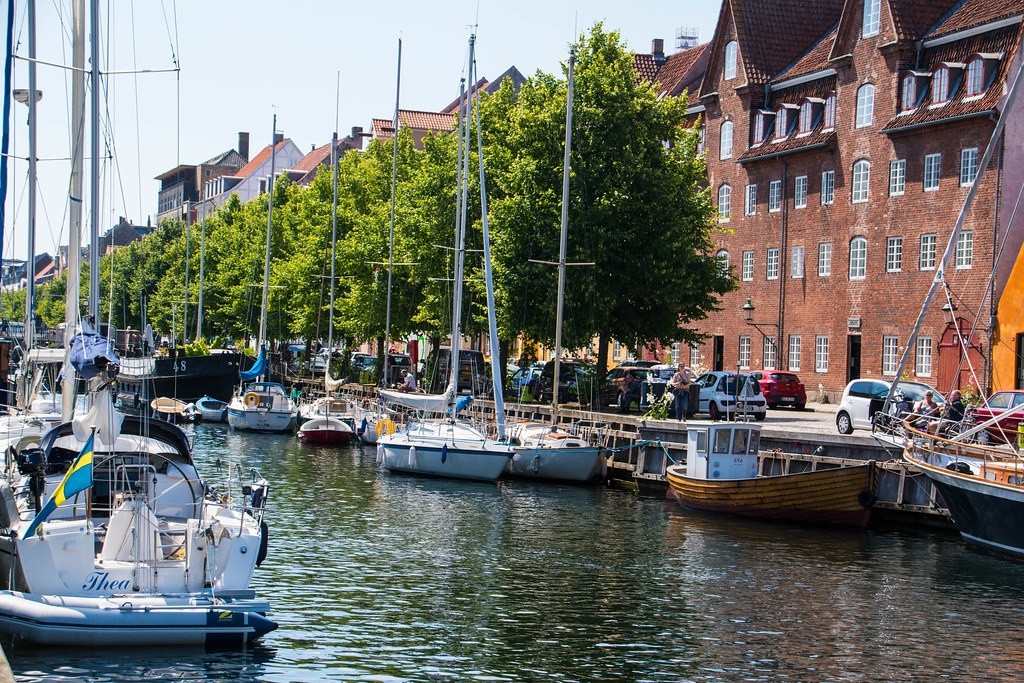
[867,61,1024,566]
[0,1,603,650]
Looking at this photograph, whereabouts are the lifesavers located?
[245,392,259,405]
[858,490,875,507]
[376,418,395,435]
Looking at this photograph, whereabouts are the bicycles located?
[870,393,912,436]
[947,395,977,444]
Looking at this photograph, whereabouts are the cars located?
[966,391,1024,449]
[692,371,767,423]
[748,369,808,410]
[834,377,946,436]
[275,334,699,414]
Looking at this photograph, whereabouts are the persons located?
[290,382,303,407]
[928,389,965,437]
[903,390,940,433]
[671,362,690,422]
[616,373,640,415]
[397,369,416,393]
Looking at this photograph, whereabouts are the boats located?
[631,470,671,495]
[665,421,879,531]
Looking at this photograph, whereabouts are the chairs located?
[731,376,743,387]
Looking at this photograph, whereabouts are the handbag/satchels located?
[673,387,684,398]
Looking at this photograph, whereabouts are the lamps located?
[940,293,985,360]
[742,298,777,352]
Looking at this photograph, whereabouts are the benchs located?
[901,404,975,438]
